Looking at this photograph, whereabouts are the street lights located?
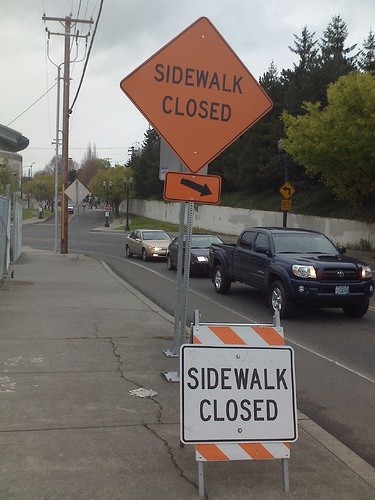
[102,180,112,227]
[124,176,133,232]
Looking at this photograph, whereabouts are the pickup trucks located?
[208,226,375,318]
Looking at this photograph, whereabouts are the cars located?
[125,229,175,262]
[166,233,225,277]
[68,204,74,213]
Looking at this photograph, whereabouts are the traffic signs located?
[122,17,275,384]
[180,309,299,497]
[64,179,91,259]
[279,182,296,227]
[157,169,223,359]
